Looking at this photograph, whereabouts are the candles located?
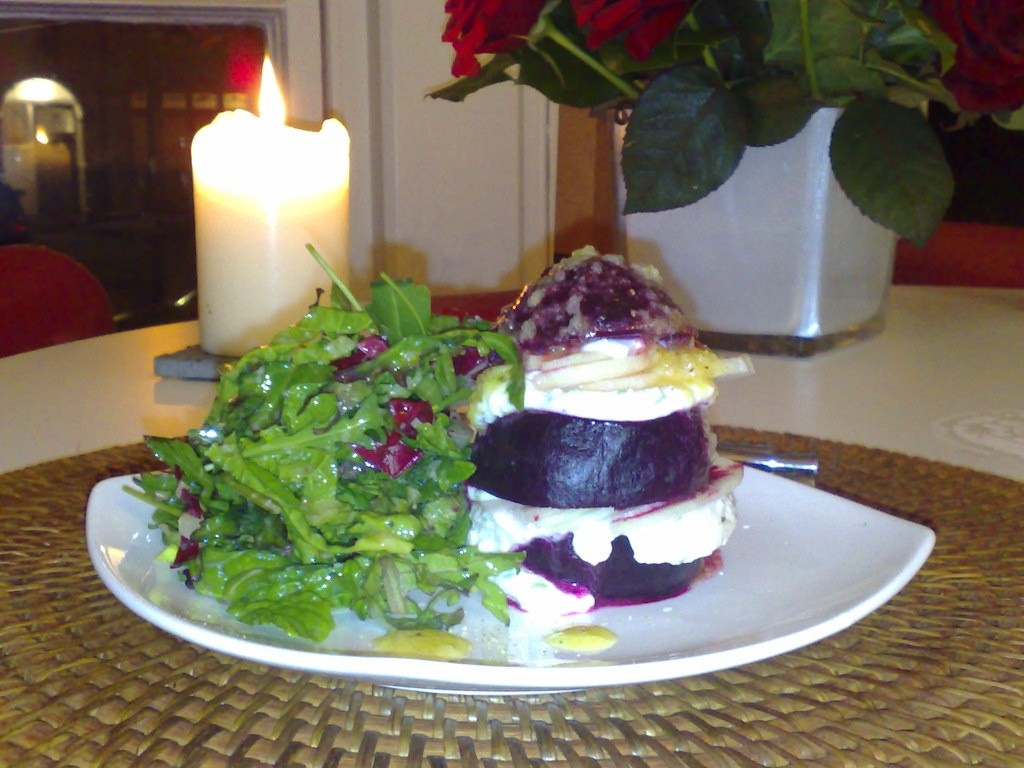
[191,53,350,355]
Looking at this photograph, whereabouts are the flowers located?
[421,0,1023,248]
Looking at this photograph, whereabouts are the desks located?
[1,288,1024,767]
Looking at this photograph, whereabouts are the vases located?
[627,107,896,336]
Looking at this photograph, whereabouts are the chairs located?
[0,245,112,360]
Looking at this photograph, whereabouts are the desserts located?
[465,243,741,618]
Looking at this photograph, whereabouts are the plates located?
[87,457,939,695]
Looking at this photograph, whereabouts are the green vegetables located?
[116,247,528,646]
